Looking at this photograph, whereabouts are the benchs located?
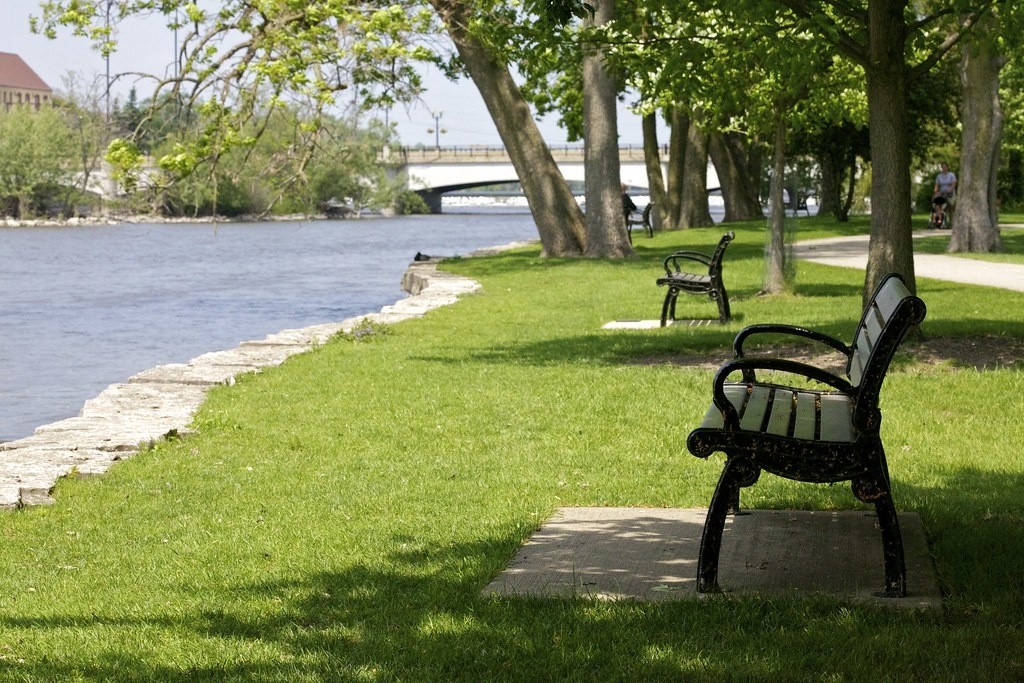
[782,194,810,219]
[652,229,739,330]
[629,200,657,238]
[681,269,932,599]
[624,205,636,247]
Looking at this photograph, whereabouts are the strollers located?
[927,194,955,229]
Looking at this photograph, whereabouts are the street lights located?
[426,111,447,145]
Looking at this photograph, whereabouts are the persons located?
[934,163,957,229]
[621,183,637,225]
[932,204,943,223]
